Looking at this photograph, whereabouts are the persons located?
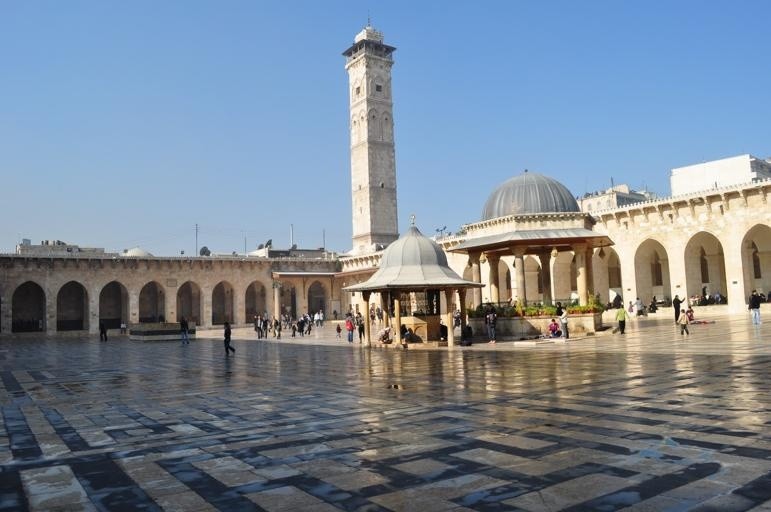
[549,302,570,338]
[613,295,628,334]
[748,292,771,324]
[703,286,708,298]
[628,295,658,316]
[180,315,190,344]
[100,319,108,342]
[224,321,237,355]
[401,303,498,344]
[689,294,727,306]
[672,295,694,336]
[121,320,128,334]
[254,309,325,339]
[337,306,393,344]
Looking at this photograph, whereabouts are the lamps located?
[598,240,605,259]
[478,251,485,264]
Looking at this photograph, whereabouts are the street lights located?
[290,224,295,249]
[435,226,447,236]
